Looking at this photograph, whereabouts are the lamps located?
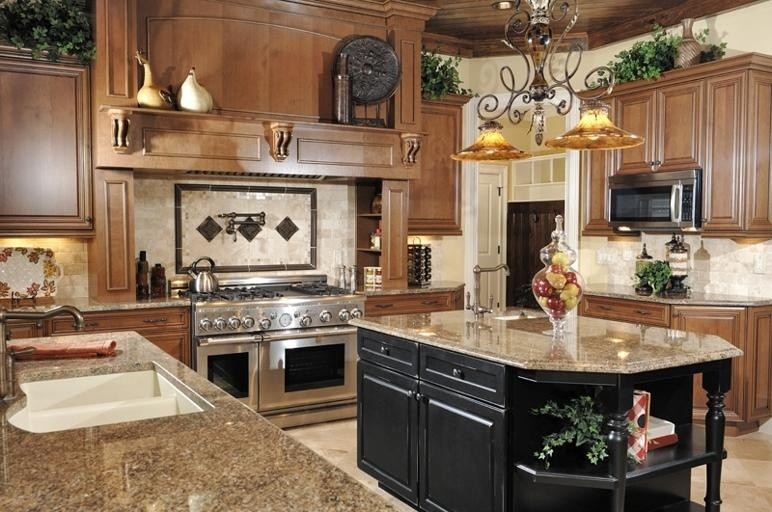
[448,0,647,163]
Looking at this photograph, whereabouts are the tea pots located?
[186,255,219,294]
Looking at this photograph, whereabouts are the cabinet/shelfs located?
[364,288,465,314]
[506,360,733,511]
[669,306,771,426]
[406,89,471,237]
[0,43,98,241]
[702,52,772,238]
[574,87,617,237]
[616,65,702,175]
[576,295,670,325]
[355,325,506,511]
[0,306,191,364]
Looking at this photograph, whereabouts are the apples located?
[534,248,582,318]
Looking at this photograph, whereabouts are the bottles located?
[151,262,166,297]
[365,267,383,285]
[338,264,357,277]
[135,250,148,299]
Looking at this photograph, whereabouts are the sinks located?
[5,361,216,435]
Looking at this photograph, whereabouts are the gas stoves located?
[171,274,368,307]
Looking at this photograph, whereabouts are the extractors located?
[185,170,327,180]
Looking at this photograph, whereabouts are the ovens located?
[196,332,259,415]
[258,324,358,415]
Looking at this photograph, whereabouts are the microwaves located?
[607,168,702,229]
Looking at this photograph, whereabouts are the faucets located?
[0,306,85,399]
